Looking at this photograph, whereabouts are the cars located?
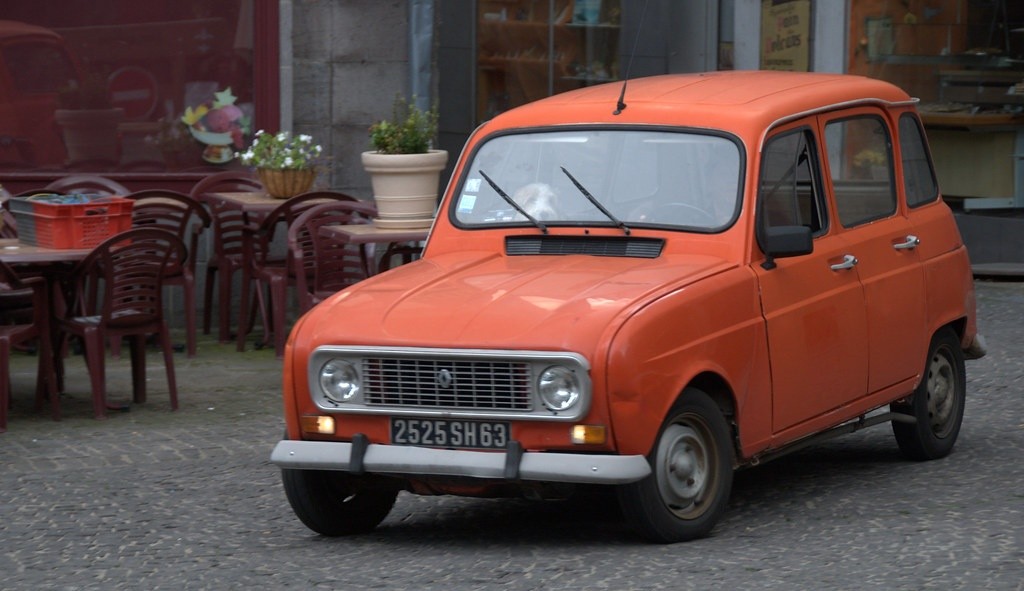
[0,20,120,178]
[271,68,988,542]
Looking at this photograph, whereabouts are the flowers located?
[234,129,338,173]
[852,149,887,179]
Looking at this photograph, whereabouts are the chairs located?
[0,168,424,434]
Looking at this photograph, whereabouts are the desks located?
[0,238,146,262]
[319,224,431,244]
[203,192,365,212]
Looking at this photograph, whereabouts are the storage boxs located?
[32,197,134,250]
[9,194,110,245]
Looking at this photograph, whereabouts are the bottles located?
[501,8,506,21]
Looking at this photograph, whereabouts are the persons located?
[556,0,620,76]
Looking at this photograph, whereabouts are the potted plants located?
[361,89,450,219]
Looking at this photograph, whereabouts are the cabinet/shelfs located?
[475,0,622,128]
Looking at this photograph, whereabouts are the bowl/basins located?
[189,123,246,163]
[484,13,500,19]
